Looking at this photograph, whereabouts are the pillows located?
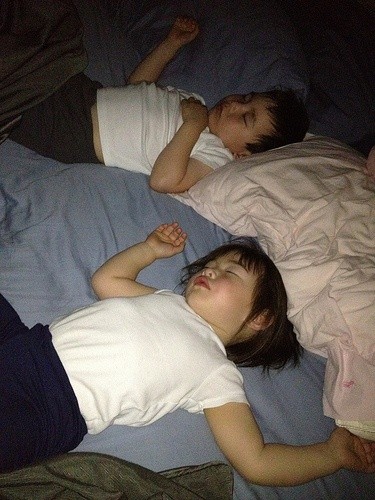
[173,129,375,445]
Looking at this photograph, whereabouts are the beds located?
[0,0,375,500]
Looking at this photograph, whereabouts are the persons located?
[7,14,309,198]
[0,219,375,487]
